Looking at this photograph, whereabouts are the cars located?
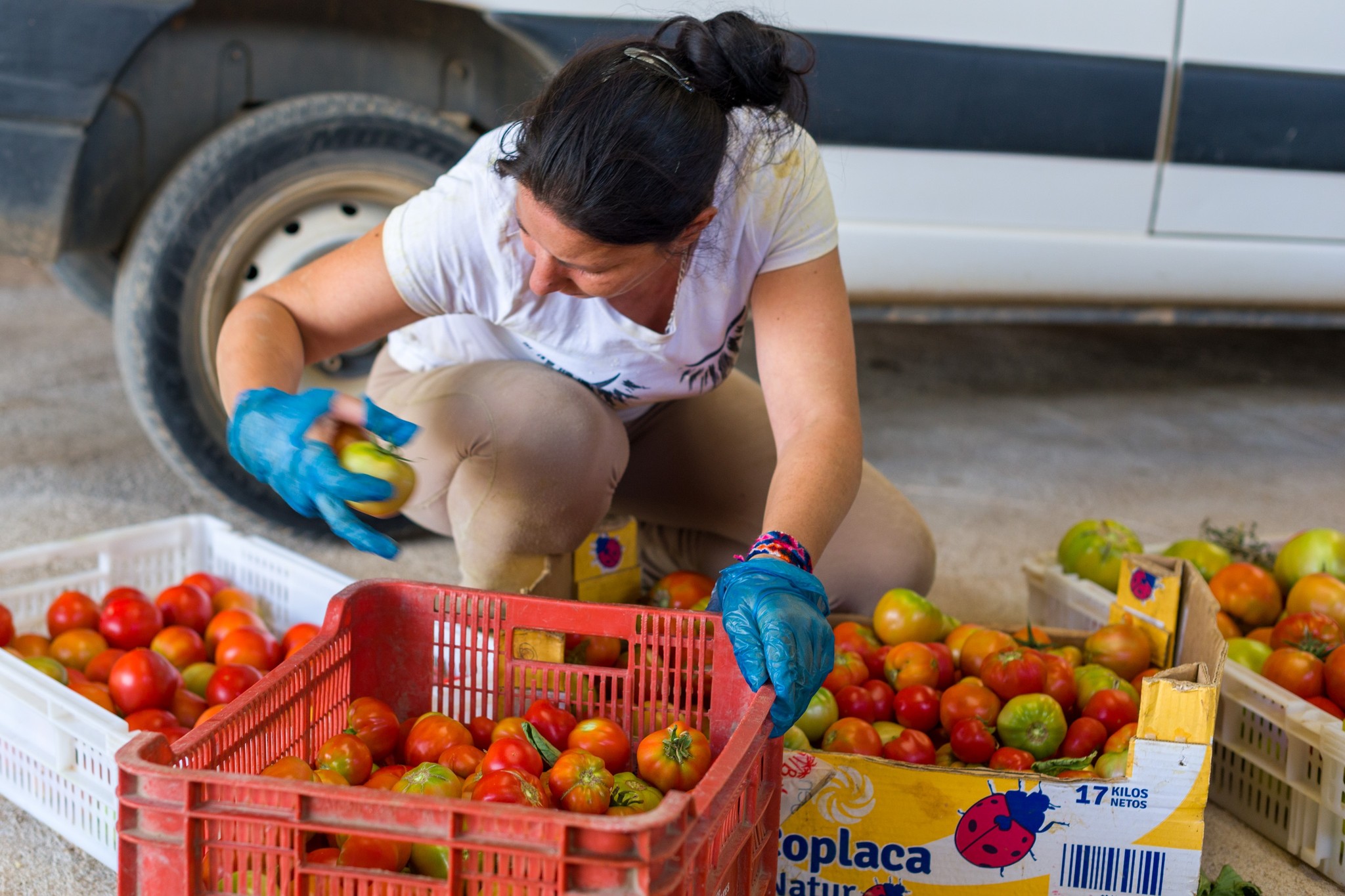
[0,0,1344,549]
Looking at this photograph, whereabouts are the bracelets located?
[732,531,814,577]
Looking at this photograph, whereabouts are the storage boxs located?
[0,516,494,864]
[116,577,778,895]
[1022,532,1345,888]
[776,556,1226,896]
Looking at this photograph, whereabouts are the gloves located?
[704,558,837,739]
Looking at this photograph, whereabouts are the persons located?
[216,9,937,740]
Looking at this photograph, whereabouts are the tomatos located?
[202,696,711,895]
[339,439,415,517]
[565,573,716,698]
[0,572,322,743]
[783,588,1162,779]
[1057,521,1345,857]
[335,423,376,452]
